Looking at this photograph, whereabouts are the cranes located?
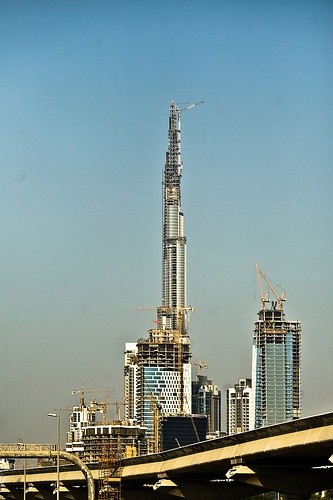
[69,385,122,424]
[149,390,163,453]
[194,359,209,380]
[124,303,196,413]
[254,261,286,310]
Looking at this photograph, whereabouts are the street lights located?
[17,442,26,500]
[47,413,60,500]
